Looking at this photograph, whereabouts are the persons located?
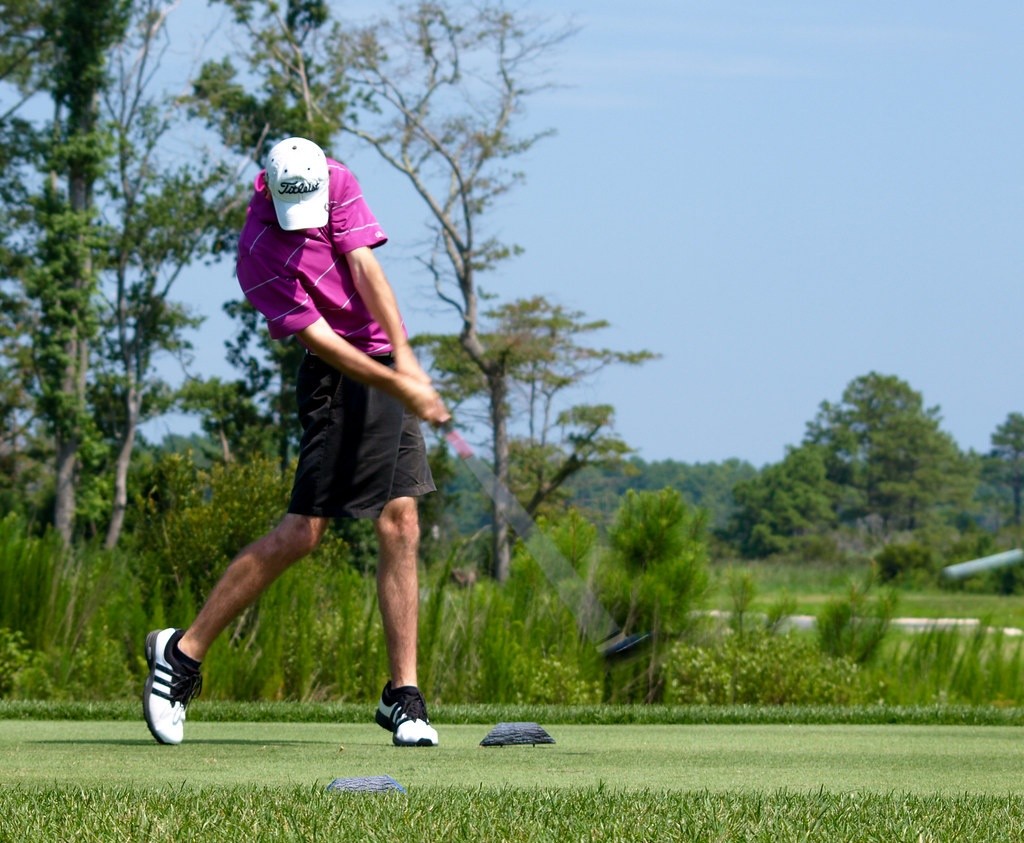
[141,136,454,746]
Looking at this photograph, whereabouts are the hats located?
[265,137,330,230]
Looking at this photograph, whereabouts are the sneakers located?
[375,679,439,746]
[142,627,204,745]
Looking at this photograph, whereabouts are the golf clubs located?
[431,412,658,666]
